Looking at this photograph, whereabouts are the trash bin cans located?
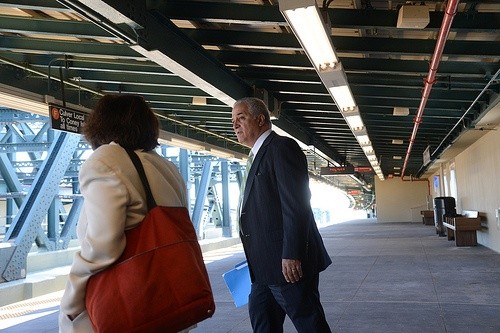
[433,196,456,237]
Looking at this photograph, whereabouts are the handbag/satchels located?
[84,144,216,333]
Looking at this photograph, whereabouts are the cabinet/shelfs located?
[421,211,434,225]
[443,210,481,247]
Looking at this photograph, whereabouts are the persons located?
[367,206,374,218]
[231,98,332,333]
[58,93,198,333]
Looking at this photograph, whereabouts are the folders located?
[222,259,252,309]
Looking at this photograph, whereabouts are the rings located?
[292,270,296,272]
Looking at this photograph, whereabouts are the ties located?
[236,151,254,235]
